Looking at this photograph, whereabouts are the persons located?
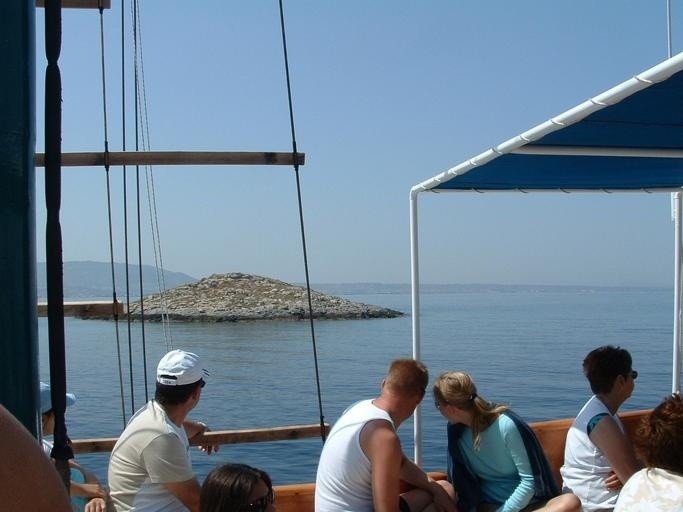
[560,347,640,512]
[105,349,220,511]
[314,360,456,511]
[199,463,277,511]
[435,371,582,512]
[613,396,683,512]
[39,380,110,512]
[0,405,74,512]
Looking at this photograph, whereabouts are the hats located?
[39,380,75,415]
[155,348,210,387]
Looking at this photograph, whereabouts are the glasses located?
[246,489,276,511]
[618,371,638,380]
